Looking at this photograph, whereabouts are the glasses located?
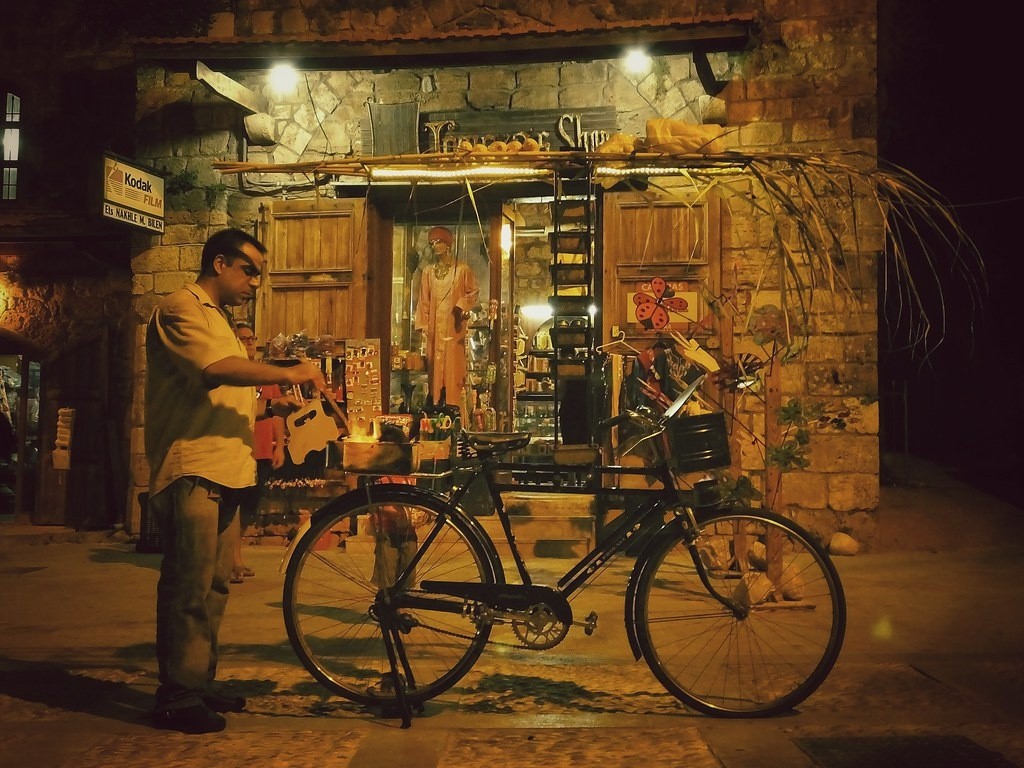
[239,336,258,344]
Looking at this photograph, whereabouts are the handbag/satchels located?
[396,506,432,529]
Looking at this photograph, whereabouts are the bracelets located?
[266,398,274,418]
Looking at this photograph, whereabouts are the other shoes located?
[240,566,255,576]
[230,568,244,582]
[202,689,245,710]
[154,704,226,733]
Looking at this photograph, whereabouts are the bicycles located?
[277,405,847,729]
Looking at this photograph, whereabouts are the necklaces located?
[435,261,451,280]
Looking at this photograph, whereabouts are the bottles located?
[486,362,496,383]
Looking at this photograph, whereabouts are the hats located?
[429,227,453,245]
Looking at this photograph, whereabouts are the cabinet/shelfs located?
[272,357,346,402]
[549,179,589,383]
[515,399,555,461]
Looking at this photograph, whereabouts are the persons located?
[229,323,285,583]
[414,227,479,432]
[144,228,324,733]
[364,425,416,589]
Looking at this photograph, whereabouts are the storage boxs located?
[326,437,419,475]
[553,441,607,469]
[409,434,452,460]
